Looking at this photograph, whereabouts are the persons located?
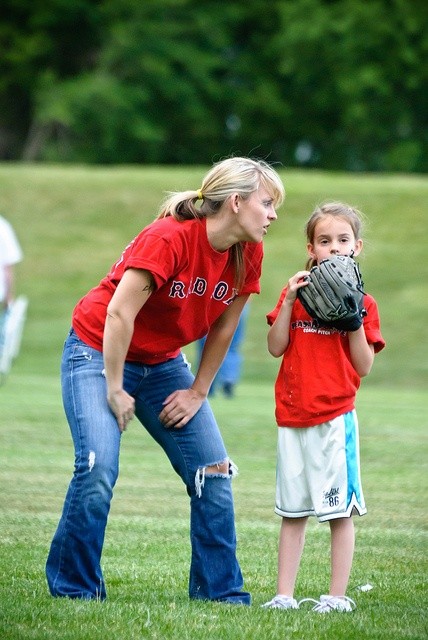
[259,202,385,614]
[45,156,286,607]
[198,300,250,398]
[0,213,23,376]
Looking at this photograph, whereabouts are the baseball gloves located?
[298,255,368,331]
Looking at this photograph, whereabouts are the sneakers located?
[260,595,299,610]
[298,594,357,612]
